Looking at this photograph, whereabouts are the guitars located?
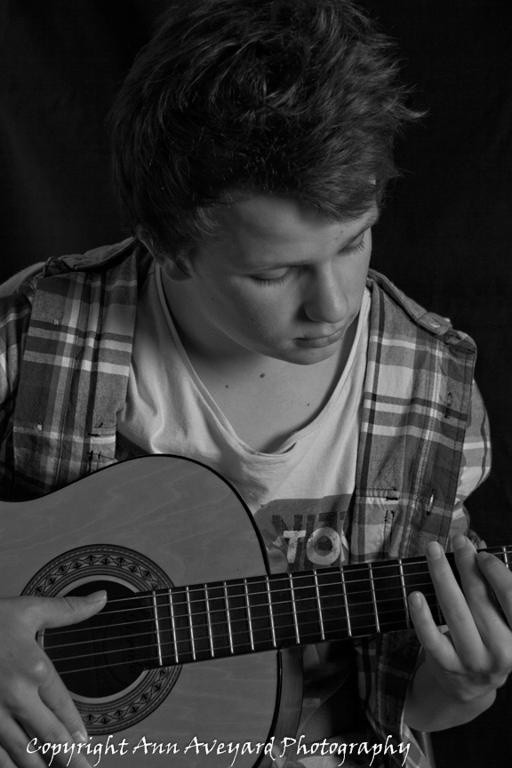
[1,453,511,768]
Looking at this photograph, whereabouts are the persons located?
[0,0,510,768]
